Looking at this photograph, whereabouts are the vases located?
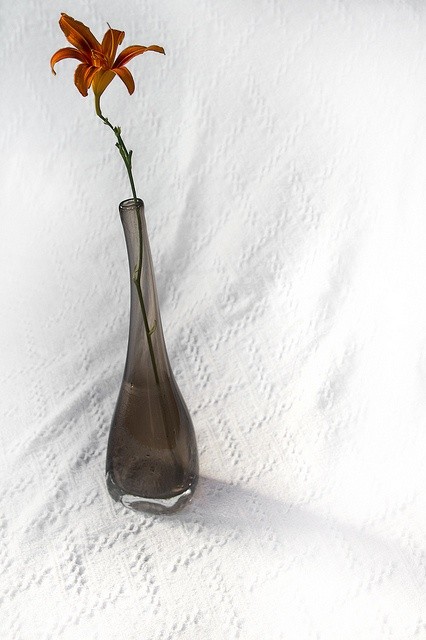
[104,197,200,517]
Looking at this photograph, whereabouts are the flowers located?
[50,12,185,485]
